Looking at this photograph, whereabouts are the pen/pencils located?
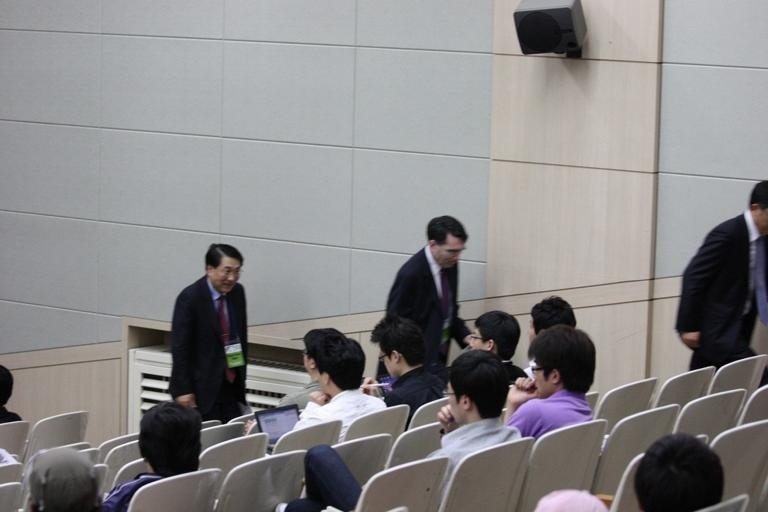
[509,380,538,390]
[360,383,390,387]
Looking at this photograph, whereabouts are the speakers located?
[513,0,587,58]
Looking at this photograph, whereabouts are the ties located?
[218,297,235,382]
[756,240,768,326]
[441,269,448,318]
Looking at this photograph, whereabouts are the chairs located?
[0,353,767,511]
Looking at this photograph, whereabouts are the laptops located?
[256,404,303,451]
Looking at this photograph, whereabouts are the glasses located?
[378,353,388,361]
[443,390,455,398]
[532,366,544,373]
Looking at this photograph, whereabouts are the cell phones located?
[377,374,393,393]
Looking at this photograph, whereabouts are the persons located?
[375,216,474,382]
[101,401,201,512]
[294,337,385,445]
[634,432,722,512]
[0,365,22,424]
[503,328,596,445]
[465,310,527,404]
[534,488,608,512]
[17,447,103,512]
[523,297,577,377]
[675,180,768,389]
[368,316,443,431]
[274,444,363,512]
[169,244,248,422]
[276,327,347,409]
[429,349,522,501]
